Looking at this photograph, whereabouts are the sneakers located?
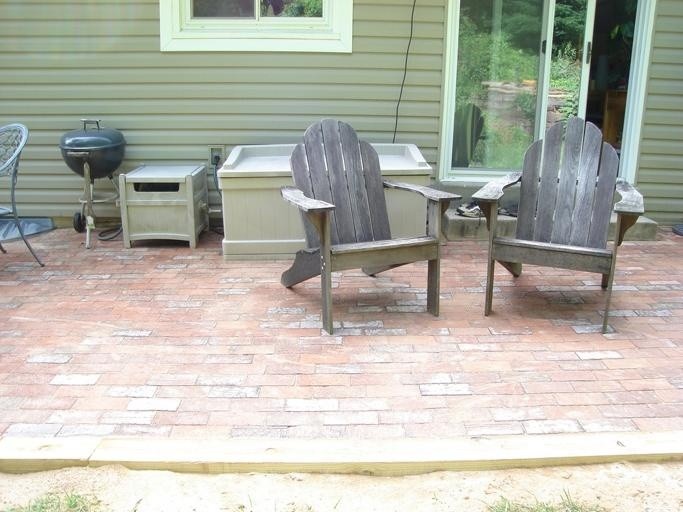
[457,202,488,217]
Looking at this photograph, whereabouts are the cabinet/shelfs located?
[119,164,210,250]
[217,144,434,259]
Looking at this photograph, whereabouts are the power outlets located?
[207,144,225,169]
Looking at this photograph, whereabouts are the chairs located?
[280,119,463,333]
[470,117,646,334]
[0,114,43,271]
